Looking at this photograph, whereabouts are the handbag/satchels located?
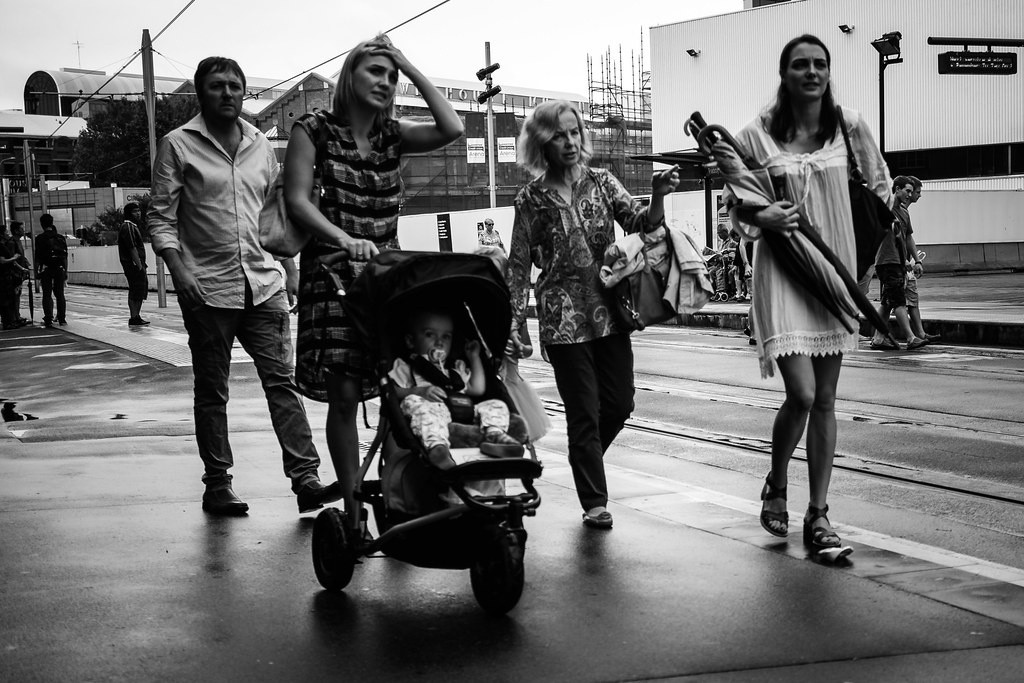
[599,205,715,332]
[836,105,896,281]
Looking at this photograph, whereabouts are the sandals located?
[804,504,841,547]
[761,471,788,537]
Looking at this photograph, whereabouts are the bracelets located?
[743,261,749,267]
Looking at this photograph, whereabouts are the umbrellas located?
[684,111,904,348]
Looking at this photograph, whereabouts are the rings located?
[357,252,364,256]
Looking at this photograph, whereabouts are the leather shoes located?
[202,489,249,515]
[297,481,343,513]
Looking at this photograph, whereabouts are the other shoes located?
[870,338,900,351]
[9,322,20,329]
[426,443,456,472]
[906,337,930,350]
[19,319,32,324]
[924,333,941,342]
[363,526,373,541]
[58,321,68,325]
[44,319,53,328]
[583,512,612,528]
[52,318,59,322]
[18,321,26,327]
[129,319,148,325]
[140,319,150,324]
[3,323,16,330]
[479,430,525,457]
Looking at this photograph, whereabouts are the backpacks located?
[41,233,68,281]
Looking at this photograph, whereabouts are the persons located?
[474,246,533,374]
[711,222,758,346]
[505,101,680,527]
[283,32,466,551]
[722,34,894,549]
[117,203,151,325]
[144,57,343,516]
[388,304,524,459]
[479,219,504,250]
[868,175,942,349]
[0,214,68,330]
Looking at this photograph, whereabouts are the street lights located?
[870,32,903,159]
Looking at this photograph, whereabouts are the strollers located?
[311,250,543,610]
